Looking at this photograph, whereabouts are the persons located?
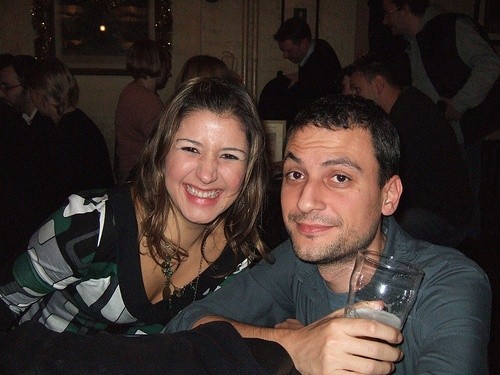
[178,56,229,97]
[274,16,344,113]
[164,95,492,375]
[0,320,301,375]
[340,54,478,250]
[1,52,55,284]
[113,38,171,183]
[25,62,118,194]
[0,75,275,343]
[383,0,500,276]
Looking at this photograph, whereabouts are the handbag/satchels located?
[0,320,301,375]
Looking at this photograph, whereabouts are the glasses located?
[385,5,403,17]
[0,83,22,93]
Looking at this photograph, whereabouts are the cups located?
[344,250,423,335]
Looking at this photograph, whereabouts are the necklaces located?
[163,225,208,322]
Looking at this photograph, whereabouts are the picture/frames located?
[31,0,172,78]
[261,119,286,162]
